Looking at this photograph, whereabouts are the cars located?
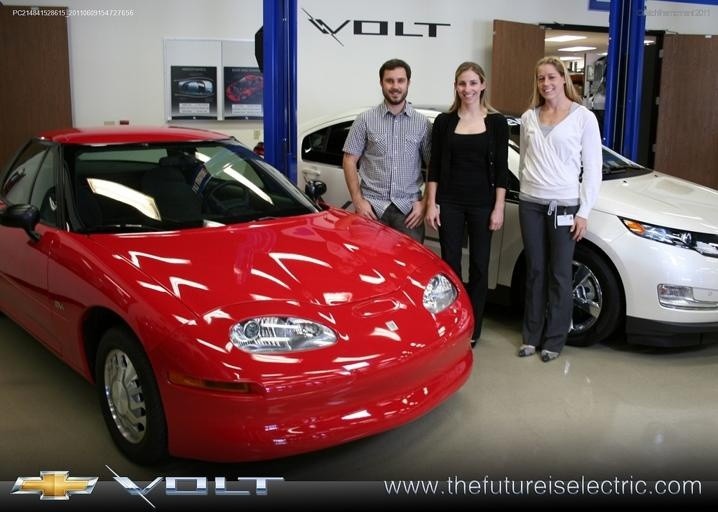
[253,101,718,351]
[0,123,480,469]
[174,79,215,103]
[226,75,263,104]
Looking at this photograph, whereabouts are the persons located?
[342,59,432,245]
[425,61,508,347]
[519,56,602,363]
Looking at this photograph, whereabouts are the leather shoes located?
[517,344,537,358]
[540,349,559,363]
